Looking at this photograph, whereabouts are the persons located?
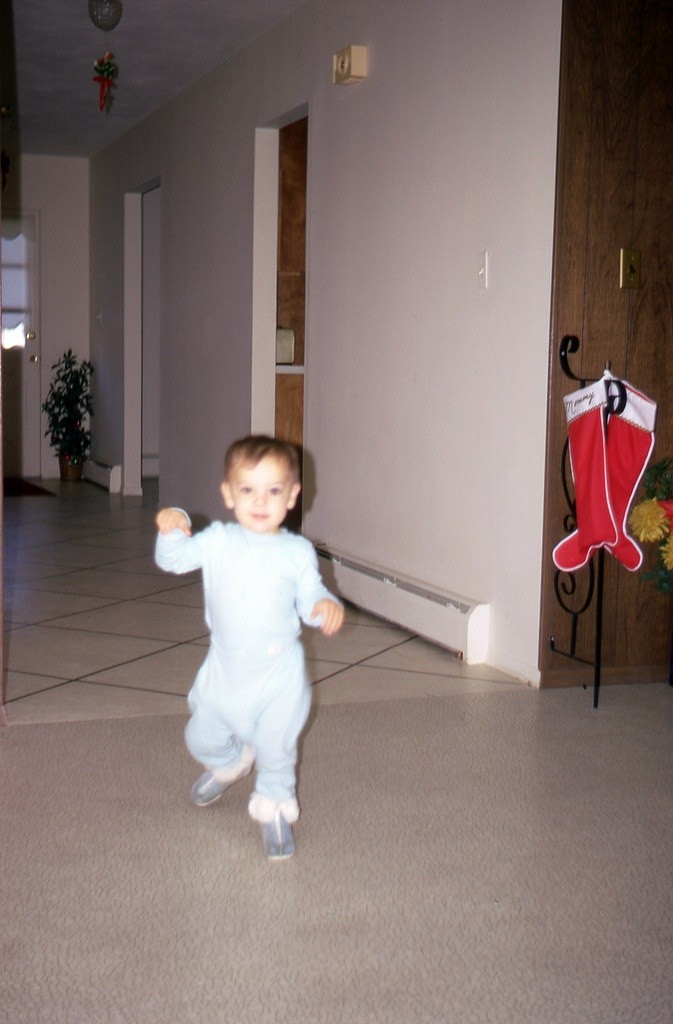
[154,433,343,856]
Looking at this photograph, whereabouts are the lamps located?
[88,0,123,33]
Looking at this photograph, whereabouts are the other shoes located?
[190,743,255,806]
[248,792,300,861]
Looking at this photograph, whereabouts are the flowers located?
[625,456,673,596]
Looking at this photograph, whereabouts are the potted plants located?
[40,349,98,482]
